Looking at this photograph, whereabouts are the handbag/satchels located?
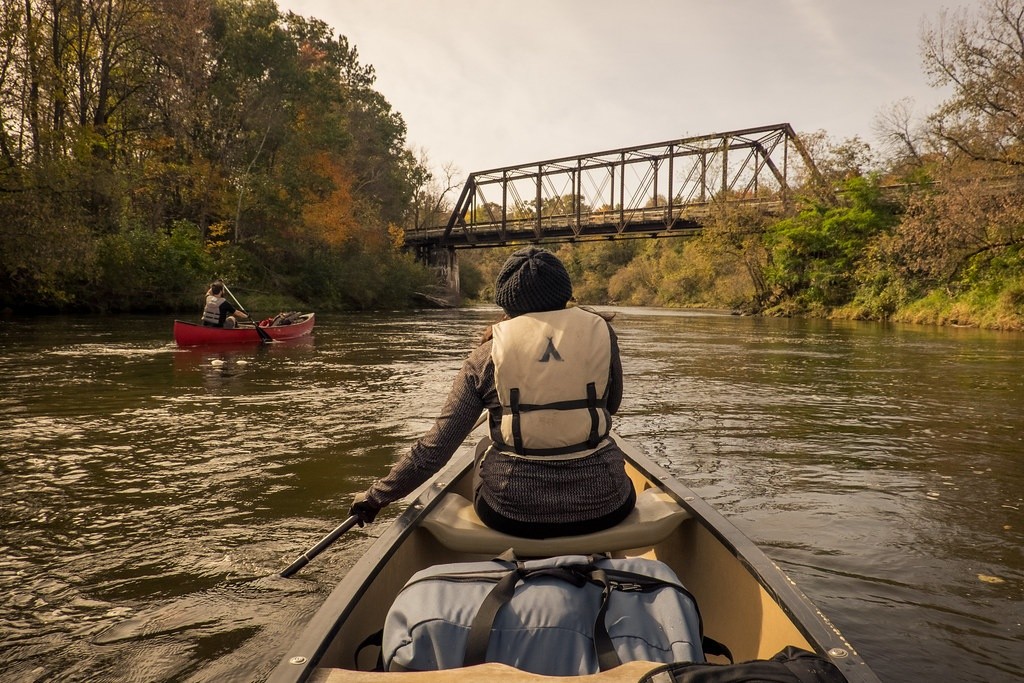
[352,546,733,668]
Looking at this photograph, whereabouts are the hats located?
[495,247,572,318]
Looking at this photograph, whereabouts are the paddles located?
[279,410,489,578]
[220,280,273,342]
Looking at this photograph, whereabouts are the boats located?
[173,312,316,346]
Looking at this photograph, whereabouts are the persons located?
[348,246,636,538]
[201,278,250,328]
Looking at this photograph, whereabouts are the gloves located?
[348,487,385,526]
